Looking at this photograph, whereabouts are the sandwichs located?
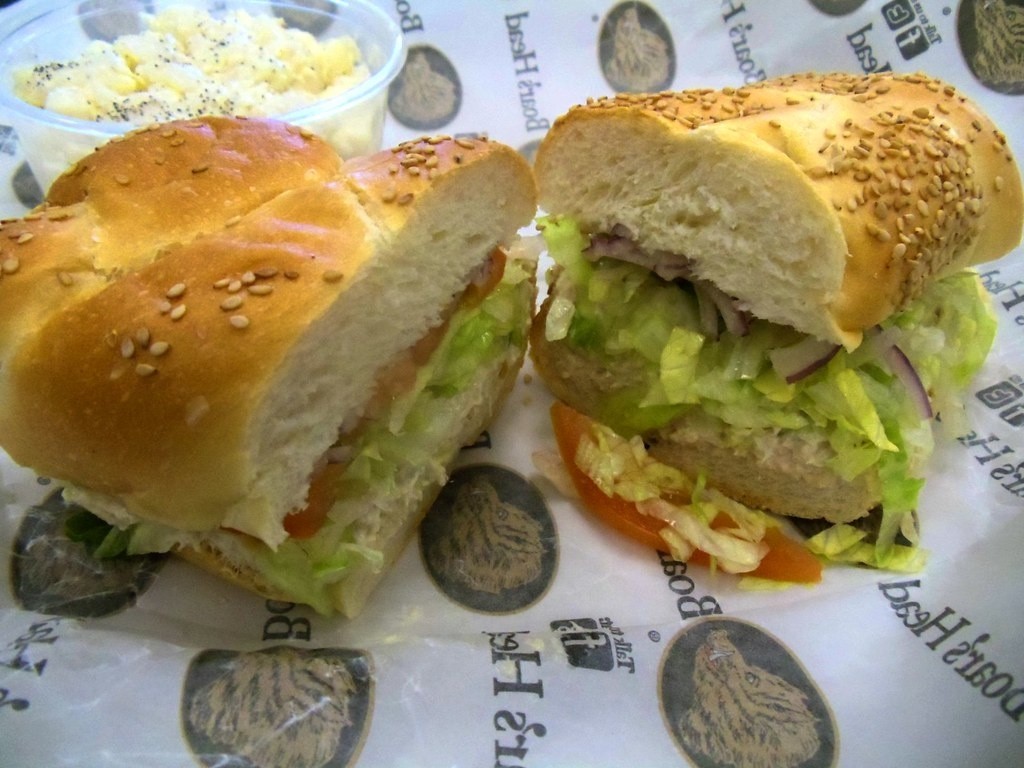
[528,75,1024,586]
[0,115,536,620]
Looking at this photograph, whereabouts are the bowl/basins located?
[0,0,408,201]
